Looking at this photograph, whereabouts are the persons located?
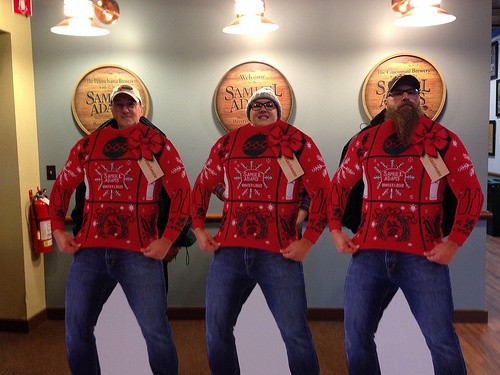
[214,87,311,225]
[69,85,197,294]
[48,123,191,375]
[190,121,330,375]
[326,117,484,375]
[339,74,457,236]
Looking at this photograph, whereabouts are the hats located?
[386,74,421,98]
[110,84,142,106]
[246,87,282,123]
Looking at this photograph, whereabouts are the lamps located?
[391,0,457,26]
[51,0,120,36]
[223,0,278,34]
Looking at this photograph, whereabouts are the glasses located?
[112,101,139,110]
[250,101,277,111]
[387,88,419,97]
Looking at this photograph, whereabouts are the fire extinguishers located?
[27,189,53,254]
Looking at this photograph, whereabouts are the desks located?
[487,175,500,237]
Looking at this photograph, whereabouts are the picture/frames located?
[490,41,498,80]
[496,79,500,117]
[488,120,496,156]
